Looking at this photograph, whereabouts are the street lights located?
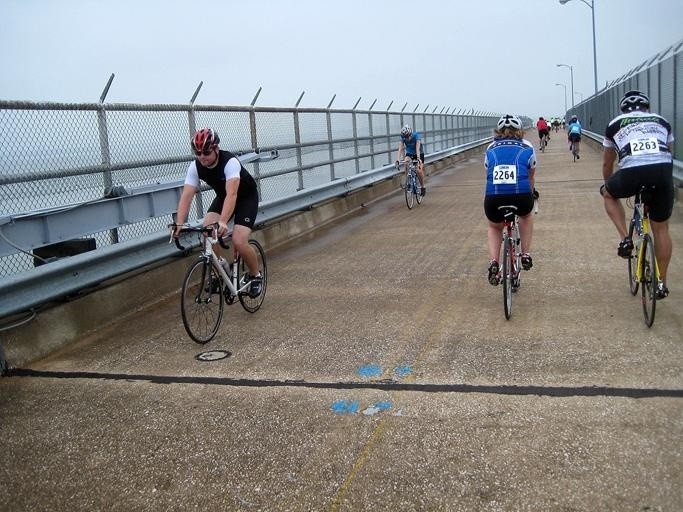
[558,0,598,95]
[555,83,568,111]
[556,64,573,109]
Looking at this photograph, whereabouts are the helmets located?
[497,113,521,131]
[400,125,412,139]
[190,128,220,150]
[620,90,650,112]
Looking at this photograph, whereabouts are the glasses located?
[194,151,212,156]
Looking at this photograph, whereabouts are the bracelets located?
[416,156,420,158]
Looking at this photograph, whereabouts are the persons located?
[394,124,426,198]
[535,113,584,161]
[483,112,538,287]
[172,125,265,299]
[599,89,675,300]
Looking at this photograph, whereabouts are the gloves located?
[532,188,539,199]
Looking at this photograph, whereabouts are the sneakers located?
[420,188,425,196]
[617,237,634,255]
[656,286,669,298]
[487,261,498,286]
[205,275,223,294]
[249,271,263,298]
[520,255,532,268]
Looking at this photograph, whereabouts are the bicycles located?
[167,222,268,344]
[395,159,424,208]
[599,181,664,329]
[539,133,549,153]
[551,124,564,132]
[568,137,578,161]
[498,189,538,319]
